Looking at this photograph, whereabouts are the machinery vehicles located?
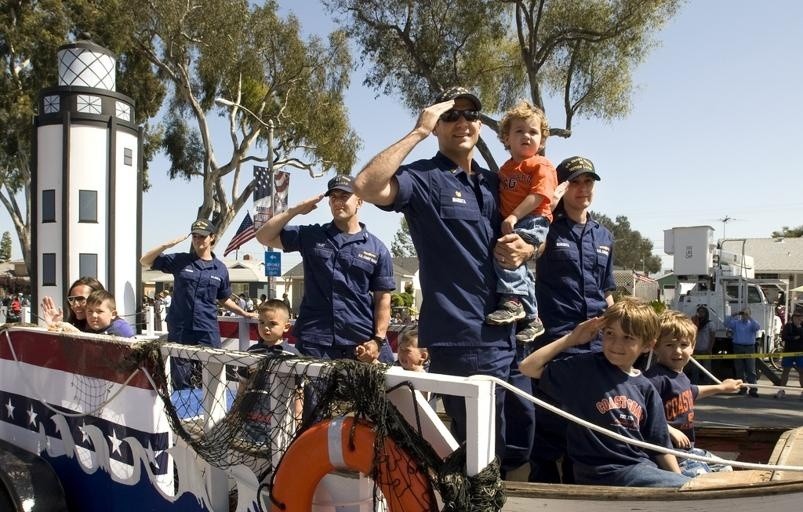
[661,223,783,362]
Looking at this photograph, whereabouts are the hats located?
[324,175,356,196]
[556,156,600,186]
[190,219,214,236]
[436,87,481,111]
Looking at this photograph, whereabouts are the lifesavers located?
[271,417,439,511]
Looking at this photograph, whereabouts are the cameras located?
[739,311,745,315]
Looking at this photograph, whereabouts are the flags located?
[223,211,258,259]
[249,165,272,230]
[274,169,290,218]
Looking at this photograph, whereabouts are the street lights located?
[214,96,276,302]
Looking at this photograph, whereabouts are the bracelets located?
[530,243,540,261]
[372,334,386,344]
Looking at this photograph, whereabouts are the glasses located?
[441,109,479,121]
[68,296,87,302]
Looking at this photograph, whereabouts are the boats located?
[171,386,803,512]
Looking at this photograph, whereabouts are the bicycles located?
[770,338,799,372]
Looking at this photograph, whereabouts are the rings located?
[501,257,504,263]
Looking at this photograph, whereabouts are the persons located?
[38,274,104,327]
[351,84,547,480]
[46,289,135,339]
[721,306,761,398]
[525,155,616,484]
[642,309,744,477]
[690,306,717,386]
[778,310,802,388]
[1,289,31,325]
[139,285,175,321]
[254,173,396,430]
[214,291,291,317]
[136,217,258,390]
[516,295,693,489]
[483,100,559,343]
[217,298,303,445]
[354,329,429,403]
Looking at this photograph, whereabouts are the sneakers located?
[486,301,526,326]
[749,392,758,398]
[516,318,545,343]
[738,391,746,395]
